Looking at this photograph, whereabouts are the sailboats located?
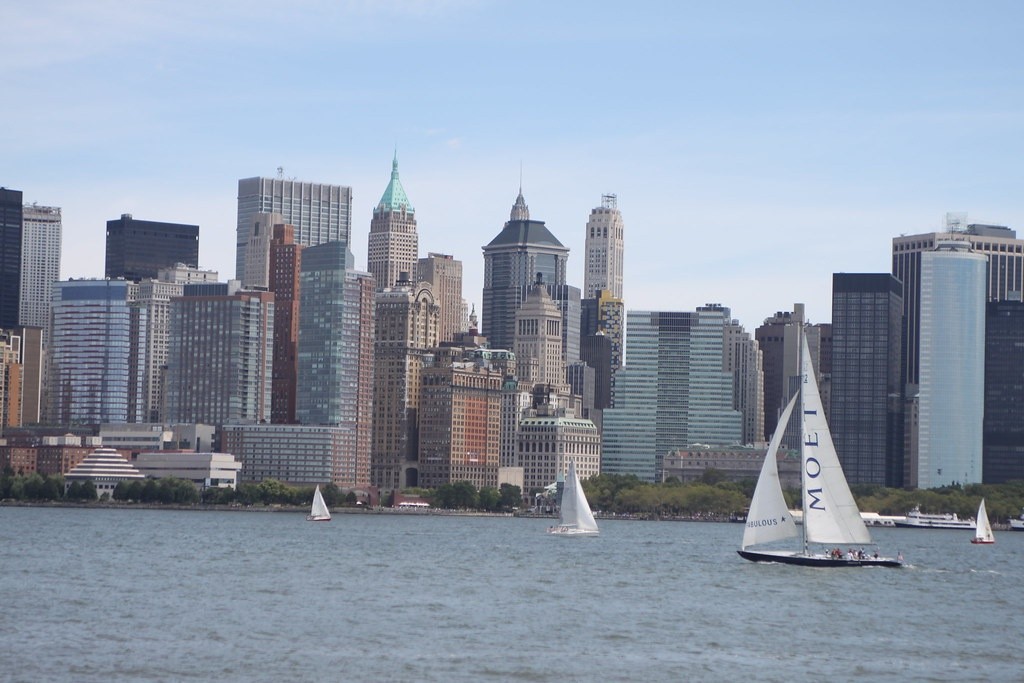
[736,330,907,569]
[307,484,334,521]
[547,461,600,538]
[970,498,996,544]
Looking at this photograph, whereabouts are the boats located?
[785,508,1024,533]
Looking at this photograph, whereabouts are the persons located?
[549,526,569,533]
[898,551,904,563]
[826,545,879,560]
